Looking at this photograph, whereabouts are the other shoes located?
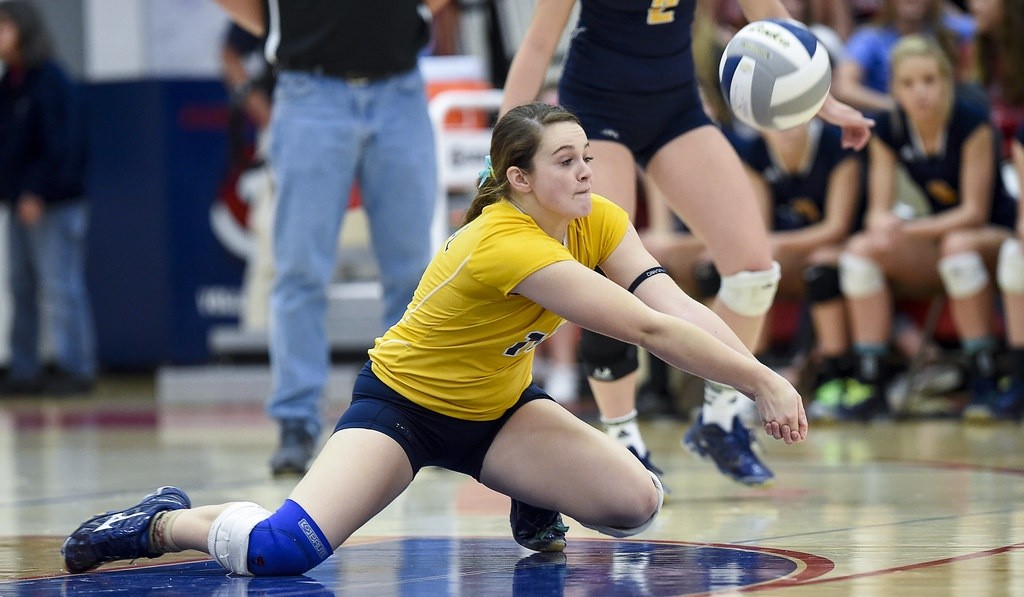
[272,417,321,476]
[963,348,1024,422]
[890,338,987,417]
[0,362,91,398]
[803,348,902,423]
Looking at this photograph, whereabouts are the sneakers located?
[508,498,570,552]
[60,486,190,577]
[512,549,567,597]
[679,409,775,487]
[627,446,664,476]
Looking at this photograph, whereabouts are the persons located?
[0,0,76,397]
[60,103,807,577]
[212,1,1024,491]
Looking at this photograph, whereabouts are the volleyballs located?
[718,17,831,134]
